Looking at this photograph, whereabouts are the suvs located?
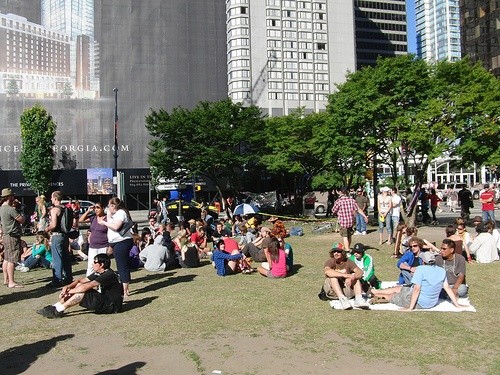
[435,182,480,201]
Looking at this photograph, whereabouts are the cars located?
[60,200,107,219]
[303,189,356,213]
[148,200,219,228]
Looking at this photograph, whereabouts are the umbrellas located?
[232,203,261,216]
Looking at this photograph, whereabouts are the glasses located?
[333,250,342,253]
[93,261,99,264]
[440,247,449,251]
[458,228,464,231]
[409,244,419,249]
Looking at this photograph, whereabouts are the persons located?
[457,184,474,221]
[36,253,124,319]
[323,242,381,310]
[0,187,26,289]
[403,185,416,208]
[492,183,500,204]
[418,187,442,225]
[446,220,500,264]
[480,183,496,226]
[44,191,74,287]
[443,192,449,202]
[129,196,293,278]
[0,196,91,272]
[372,223,468,312]
[79,197,134,299]
[376,185,402,244]
[331,185,369,255]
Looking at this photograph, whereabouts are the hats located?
[355,243,365,252]
[419,252,435,264]
[357,188,362,192]
[329,242,344,251]
[0,188,16,197]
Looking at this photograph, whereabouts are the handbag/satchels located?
[116,209,134,235]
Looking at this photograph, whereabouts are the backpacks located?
[184,246,200,268]
[56,205,73,233]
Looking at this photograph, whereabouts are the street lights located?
[112,87,119,196]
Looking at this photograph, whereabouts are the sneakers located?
[45,308,63,319]
[341,286,375,309]
[36,305,52,314]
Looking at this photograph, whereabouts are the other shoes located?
[2,263,29,288]
[61,278,73,286]
[242,267,255,274]
[394,251,400,258]
[46,280,60,288]
[353,231,361,235]
[387,240,392,246]
[362,230,366,235]
[380,240,383,244]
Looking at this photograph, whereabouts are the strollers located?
[21,213,38,235]
